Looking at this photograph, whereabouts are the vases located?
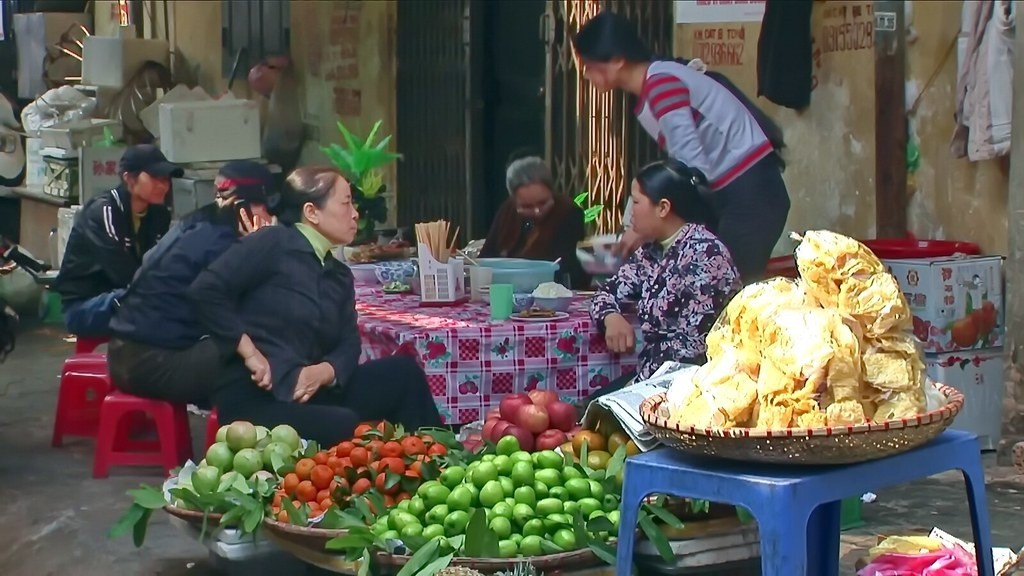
[347,214,376,246]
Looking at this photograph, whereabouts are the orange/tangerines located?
[273,425,447,523]
[559,430,640,470]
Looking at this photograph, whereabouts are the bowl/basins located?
[477,287,492,305]
[533,296,572,312]
[511,293,534,313]
[576,240,628,274]
[349,259,415,286]
[463,258,560,297]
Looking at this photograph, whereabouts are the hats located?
[214,161,272,194]
[119,143,185,179]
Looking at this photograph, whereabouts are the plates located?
[509,311,569,321]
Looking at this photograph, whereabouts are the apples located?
[952,301,997,347]
[462,389,582,452]
[371,435,620,559]
[191,421,303,495]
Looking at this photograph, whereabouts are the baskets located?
[262,515,352,553]
[375,538,619,569]
[640,382,965,461]
[162,502,238,528]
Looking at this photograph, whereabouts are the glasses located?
[515,202,553,214]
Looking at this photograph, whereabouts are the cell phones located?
[233,198,254,231]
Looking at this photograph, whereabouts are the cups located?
[467,266,514,319]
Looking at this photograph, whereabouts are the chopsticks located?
[414,219,461,263]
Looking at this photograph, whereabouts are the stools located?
[614,428,996,576]
[50,336,221,481]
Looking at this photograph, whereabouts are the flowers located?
[319,117,403,224]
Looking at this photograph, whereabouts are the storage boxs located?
[43,114,123,149]
[13,11,93,99]
[82,38,169,89]
[157,98,261,164]
[39,156,80,199]
[25,136,65,188]
[876,254,1004,453]
[78,144,130,206]
[171,168,215,221]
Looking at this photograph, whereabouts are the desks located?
[346,278,645,435]
[12,186,79,270]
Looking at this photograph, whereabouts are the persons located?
[477,154,585,292]
[574,159,744,427]
[56,143,446,452]
[570,10,791,285]
